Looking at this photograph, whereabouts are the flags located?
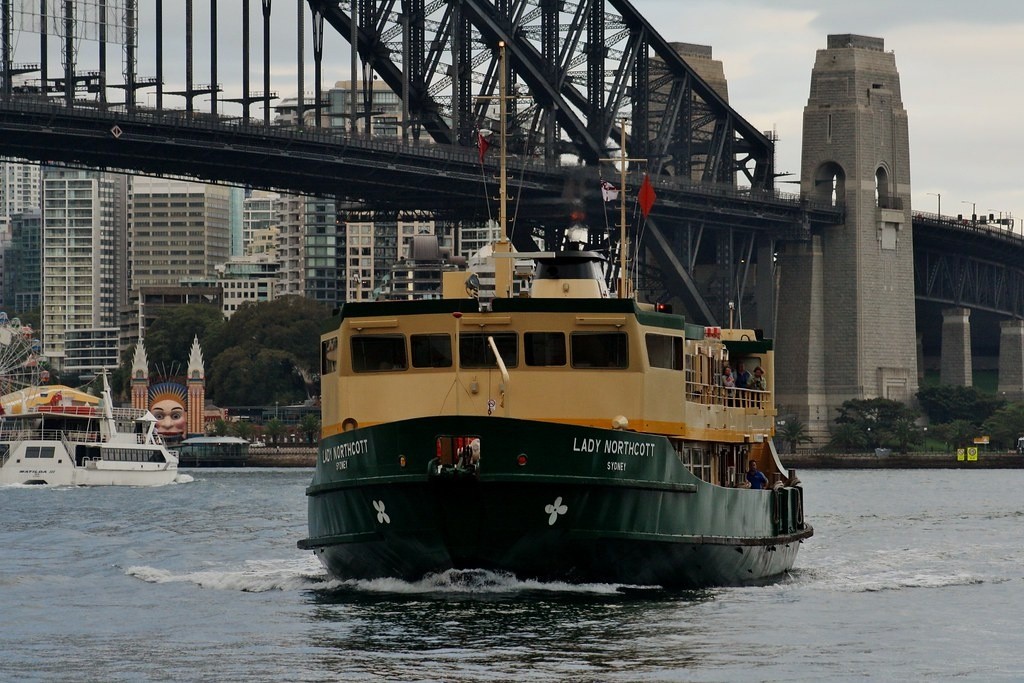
[600,180,619,202]
[636,173,657,218]
[478,134,491,164]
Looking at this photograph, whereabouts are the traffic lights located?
[958,215,962,220]
[972,215,976,220]
[990,214,993,220]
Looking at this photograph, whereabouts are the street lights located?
[1012,217,1023,240]
[962,201,975,231]
[926,192,940,219]
[988,209,1002,233]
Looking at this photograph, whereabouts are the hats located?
[753,366,764,374]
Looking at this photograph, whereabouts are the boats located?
[297,42,815,589]
[0,366,179,487]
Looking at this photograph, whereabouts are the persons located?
[943,438,1009,455]
[747,366,767,410]
[735,363,752,408]
[746,460,769,490]
[721,367,735,407]
